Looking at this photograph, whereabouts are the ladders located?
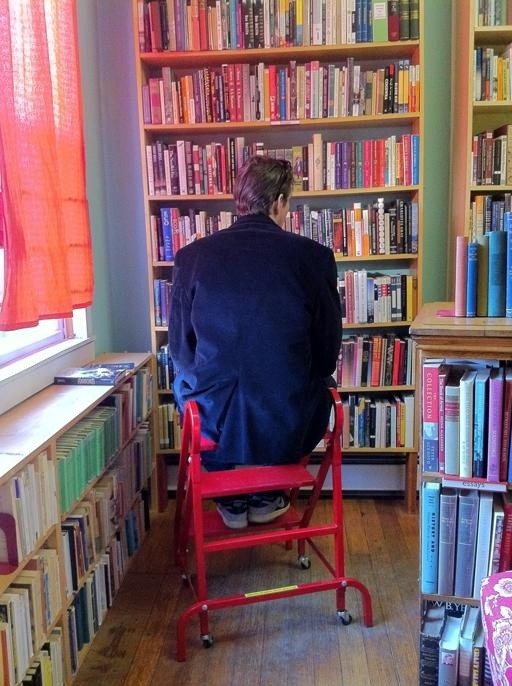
[173,386,373,665]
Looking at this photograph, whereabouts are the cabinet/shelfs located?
[446,0,512,294]
[131,0,426,517]
[402,335,512,686]
[0,351,165,686]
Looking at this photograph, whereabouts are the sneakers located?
[248,494,291,524]
[214,498,249,530]
